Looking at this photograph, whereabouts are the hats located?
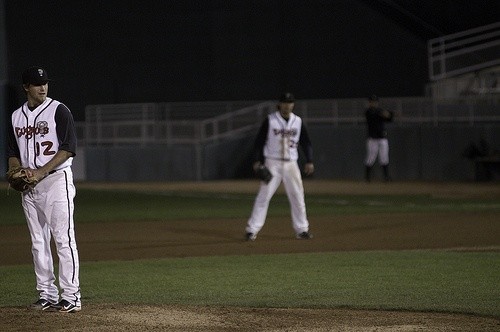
[280,92,294,102]
[23,66,56,85]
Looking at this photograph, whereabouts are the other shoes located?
[366,167,371,181]
[296,232,314,240]
[242,232,257,241]
[384,166,391,181]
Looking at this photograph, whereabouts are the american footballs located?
[9,168,33,190]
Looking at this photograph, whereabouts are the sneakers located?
[27,299,58,310]
[49,299,82,313]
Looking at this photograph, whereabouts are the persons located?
[6,65,82,312]
[242,92,315,241]
[364,93,394,183]
